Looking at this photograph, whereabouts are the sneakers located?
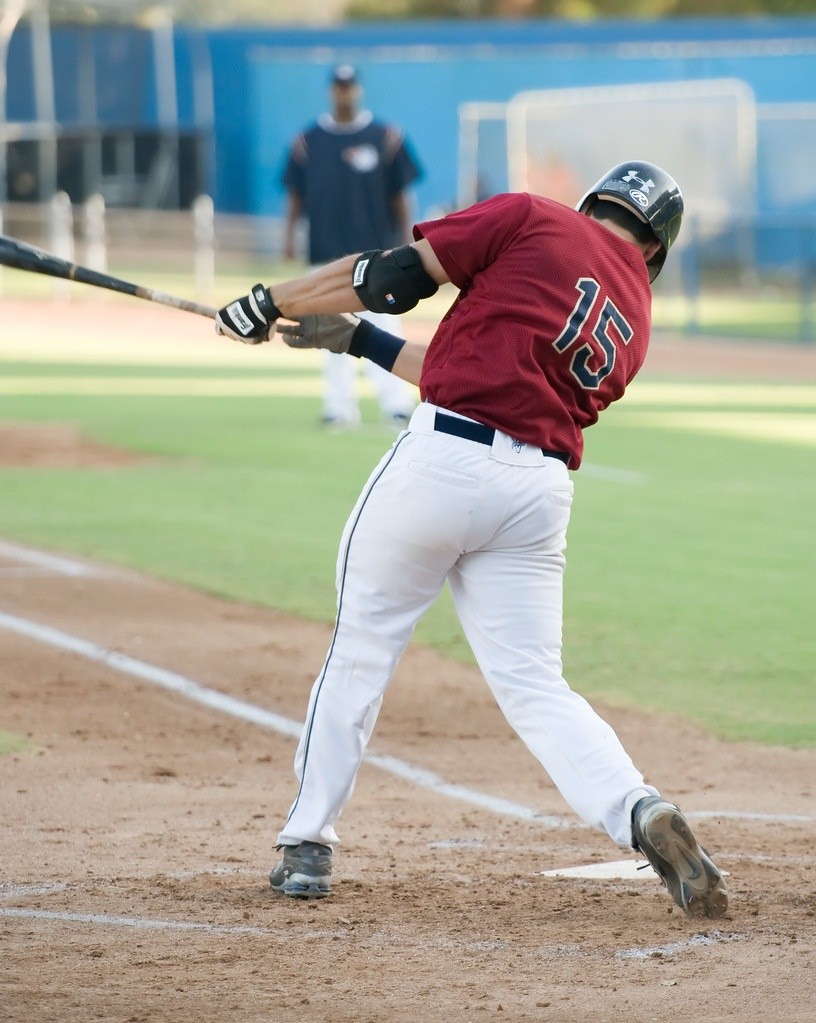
[629,795,733,918]
[267,840,336,900]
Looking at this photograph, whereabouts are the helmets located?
[573,158,686,283]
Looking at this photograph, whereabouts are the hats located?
[328,64,360,87]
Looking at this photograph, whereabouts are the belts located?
[433,412,572,466]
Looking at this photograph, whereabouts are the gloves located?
[274,311,363,355]
[212,282,284,345]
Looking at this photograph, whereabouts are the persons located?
[212,158,729,916]
[281,65,429,432]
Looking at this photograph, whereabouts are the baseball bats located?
[0,234,278,343]
